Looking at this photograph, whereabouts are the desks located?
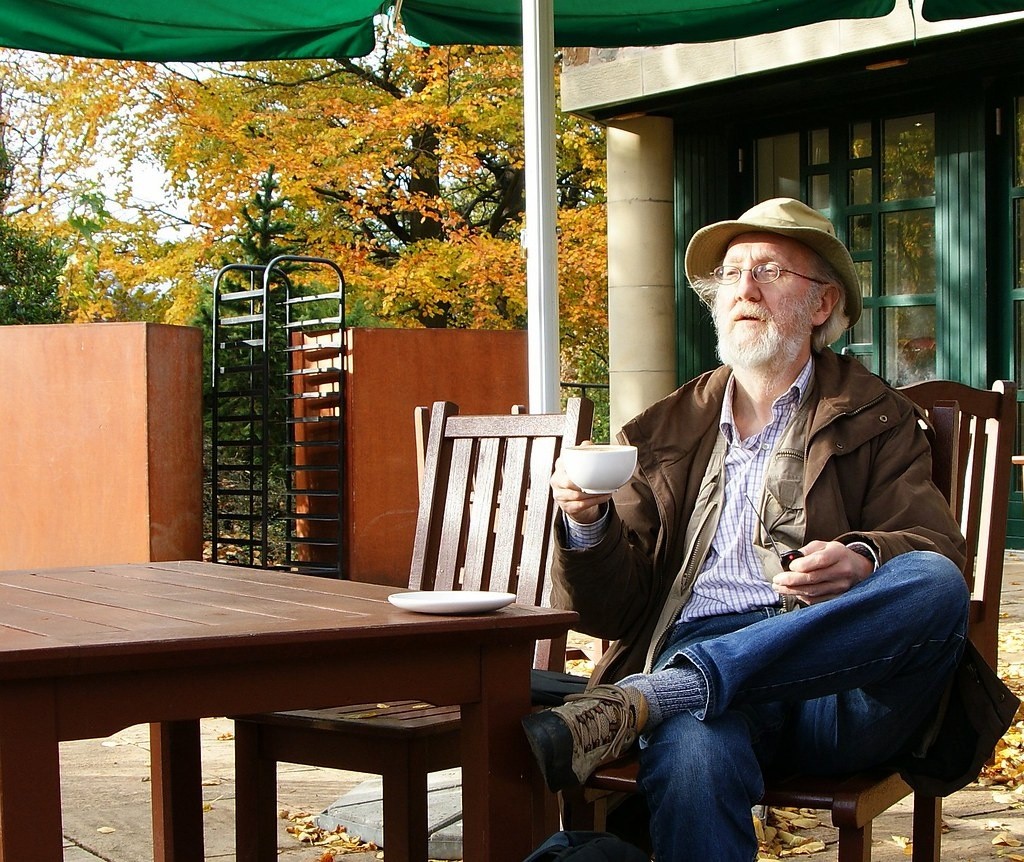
[0,561,578,862]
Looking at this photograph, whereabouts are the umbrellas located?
[1,0,1024,668]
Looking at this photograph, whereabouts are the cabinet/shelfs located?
[212,255,345,581]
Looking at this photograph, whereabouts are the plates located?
[388,589,514,614]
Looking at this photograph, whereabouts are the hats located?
[684,197,862,330]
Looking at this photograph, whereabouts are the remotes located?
[743,492,805,573]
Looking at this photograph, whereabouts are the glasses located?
[709,263,829,285]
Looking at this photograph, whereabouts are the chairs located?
[414,404,527,590]
[562,379,1017,862]
[228,397,594,862]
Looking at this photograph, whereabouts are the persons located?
[522,197,1018,862]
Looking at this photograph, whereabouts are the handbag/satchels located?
[530,669,590,706]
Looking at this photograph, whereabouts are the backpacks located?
[521,830,650,862]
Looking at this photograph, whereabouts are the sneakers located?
[521,684,648,794]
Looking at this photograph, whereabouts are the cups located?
[561,444,636,494]
